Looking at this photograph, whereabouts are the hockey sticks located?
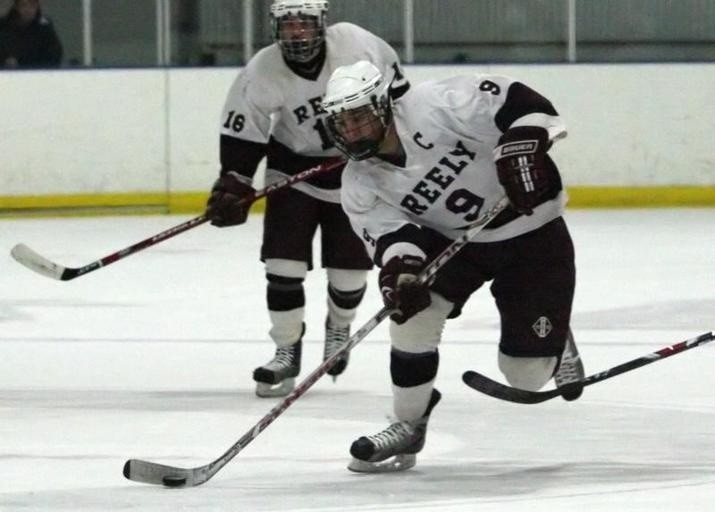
[462,330,715,405]
[122,197,508,488]
[11,156,354,280]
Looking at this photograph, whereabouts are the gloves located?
[492,124,564,216]
[204,174,256,229]
[377,253,437,326]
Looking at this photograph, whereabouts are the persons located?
[321,58,587,465]
[206,0,410,387]
[1,0,65,69]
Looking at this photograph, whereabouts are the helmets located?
[269,0,330,64]
[320,59,394,163]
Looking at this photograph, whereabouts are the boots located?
[349,387,443,463]
[252,321,307,386]
[322,312,352,377]
[550,325,585,402]
